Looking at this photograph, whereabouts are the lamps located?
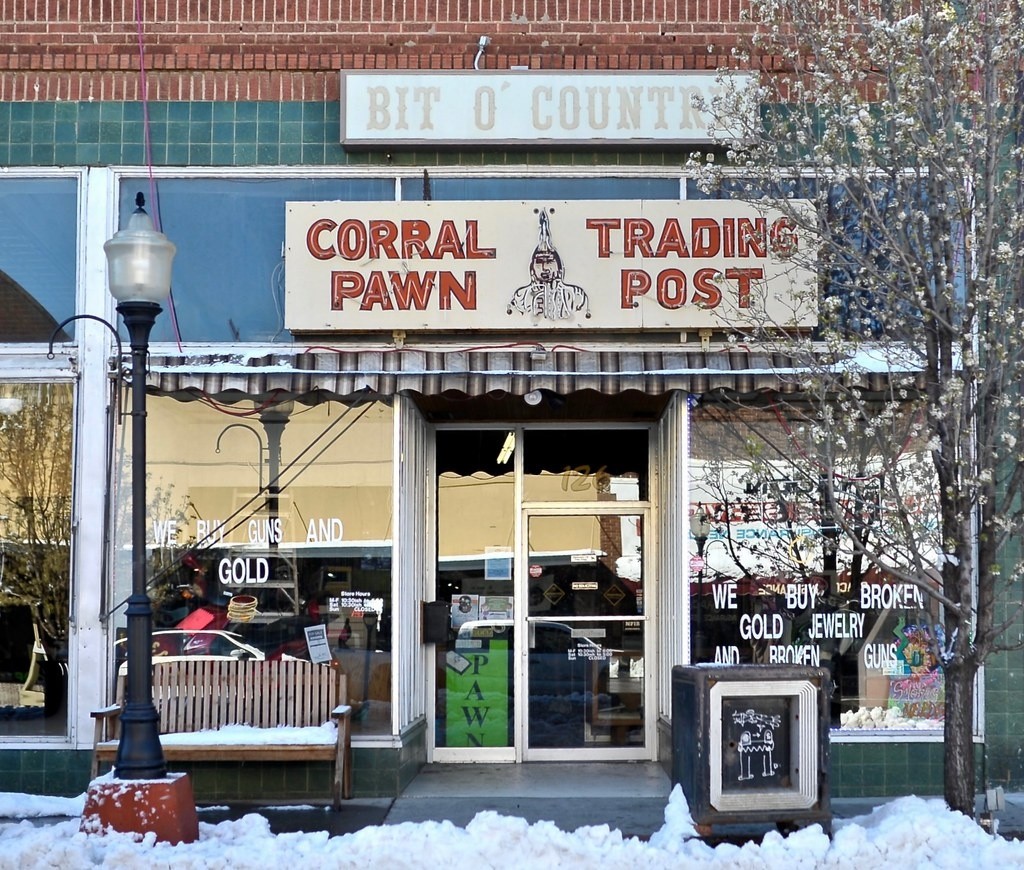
[525,389,543,406]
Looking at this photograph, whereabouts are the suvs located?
[225,581,295,624]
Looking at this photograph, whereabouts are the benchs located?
[90,654,353,813]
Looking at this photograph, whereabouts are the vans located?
[454,617,612,697]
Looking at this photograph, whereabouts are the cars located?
[105,629,335,711]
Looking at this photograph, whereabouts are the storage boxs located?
[670,666,833,837]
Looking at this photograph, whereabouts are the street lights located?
[102,191,179,778]
